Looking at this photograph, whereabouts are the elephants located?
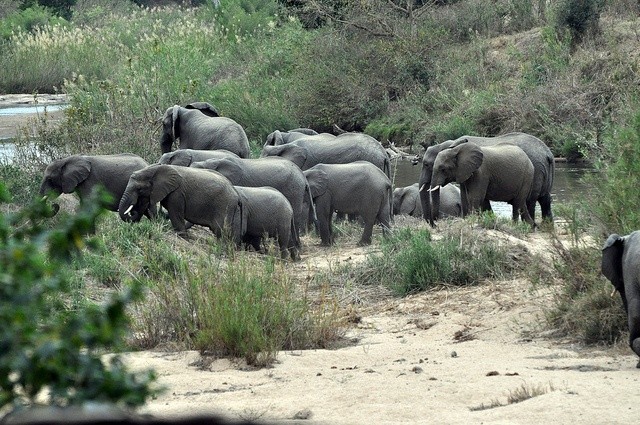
[389,182,464,220]
[36,153,157,222]
[190,155,319,255]
[418,132,556,229]
[426,138,536,233]
[120,162,243,245]
[185,186,301,264]
[159,101,250,158]
[303,160,395,248]
[259,128,336,159]
[601,230,639,368]
[263,132,392,237]
[156,149,241,211]
